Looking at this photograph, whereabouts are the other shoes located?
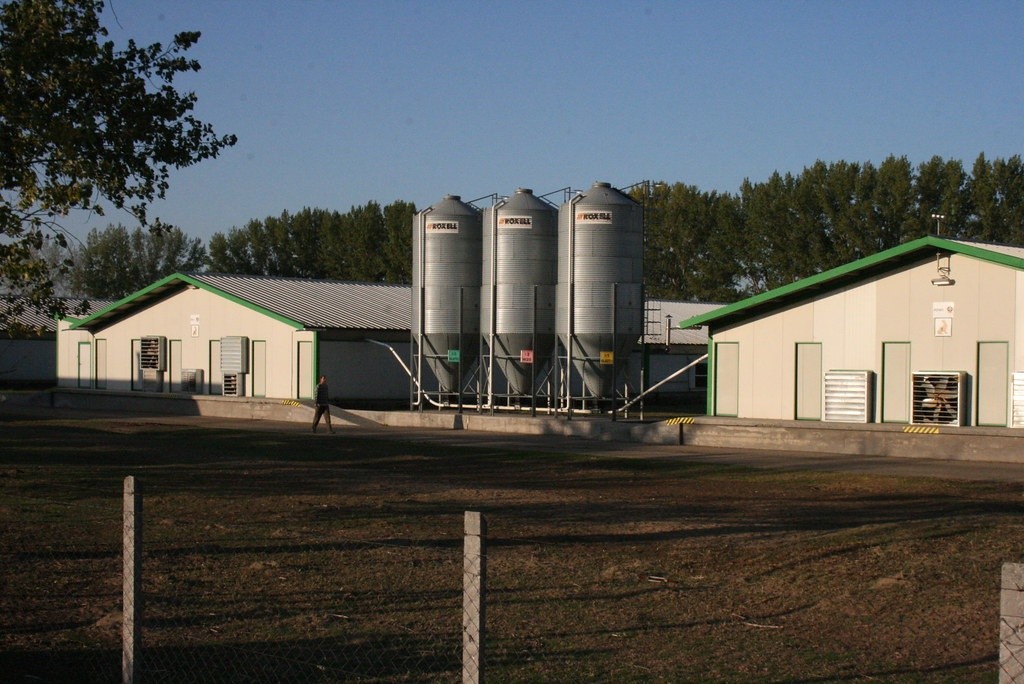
[311,428,316,433]
[327,430,335,434]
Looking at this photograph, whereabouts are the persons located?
[311,374,336,434]
